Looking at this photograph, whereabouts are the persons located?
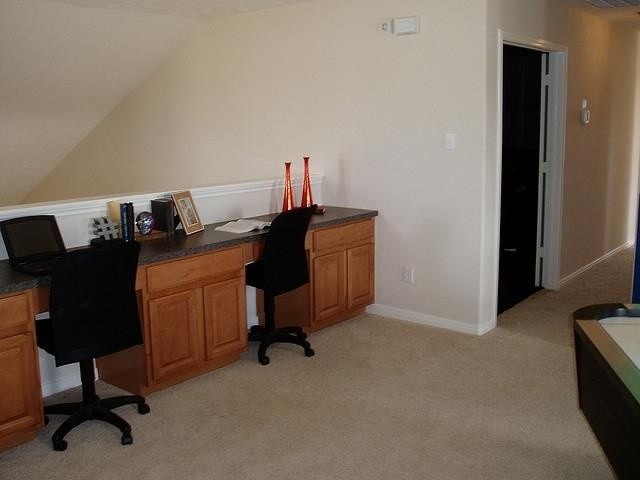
[180,200,197,225]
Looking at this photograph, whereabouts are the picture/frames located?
[172,191,205,235]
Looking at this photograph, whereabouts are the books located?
[215,219,272,234]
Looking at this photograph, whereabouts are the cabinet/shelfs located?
[313,242,375,321]
[0,331,45,451]
[148,276,248,382]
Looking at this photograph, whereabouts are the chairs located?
[246,204,318,364]
[36,242,150,451]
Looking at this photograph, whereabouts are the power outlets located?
[445,134,456,151]
[402,266,414,284]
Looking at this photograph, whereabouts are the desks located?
[0,206,379,295]
